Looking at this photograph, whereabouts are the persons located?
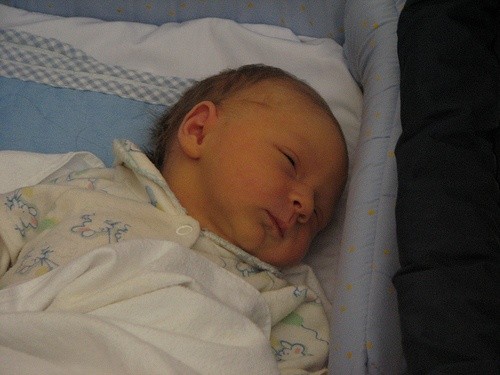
[1,63,346,375]
